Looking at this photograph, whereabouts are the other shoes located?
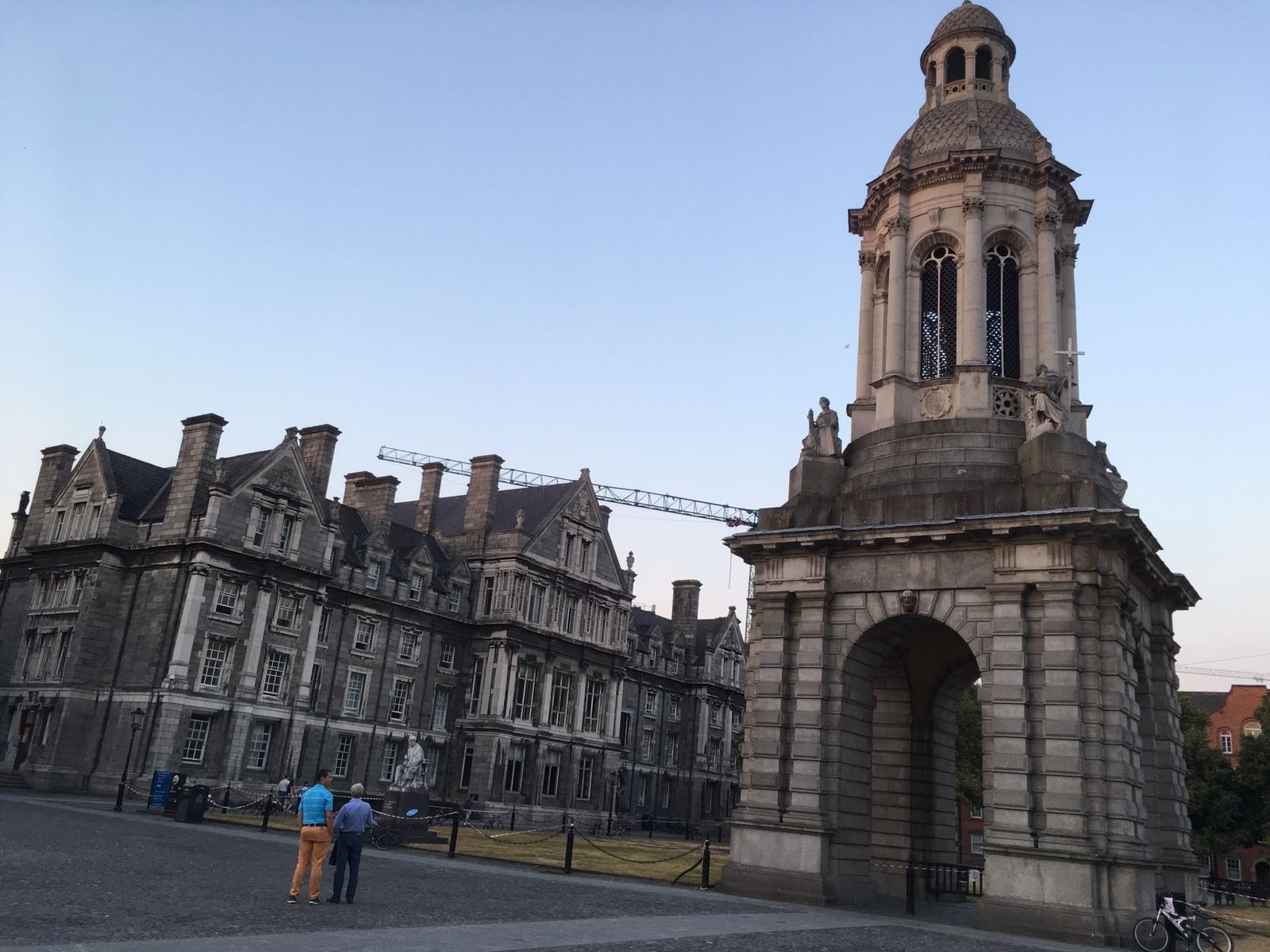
[348,900,356,903]
[324,897,339,903]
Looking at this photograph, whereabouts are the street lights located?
[113,707,146,812]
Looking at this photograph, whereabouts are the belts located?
[342,831,363,835]
[303,823,328,826]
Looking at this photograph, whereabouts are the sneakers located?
[286,896,299,903]
[309,898,320,905]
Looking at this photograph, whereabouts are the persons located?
[286,769,334,905]
[801,397,841,458]
[388,735,425,787]
[1025,363,1074,431]
[326,783,373,904]
[1094,441,1128,503]
[463,793,474,827]
[276,773,309,816]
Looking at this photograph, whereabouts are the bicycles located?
[611,824,631,837]
[482,814,504,831]
[586,823,605,837]
[659,817,704,841]
[365,822,397,850]
[1133,894,1236,952]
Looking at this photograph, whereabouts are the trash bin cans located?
[174,783,210,822]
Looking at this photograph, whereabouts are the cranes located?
[376,447,761,639]
